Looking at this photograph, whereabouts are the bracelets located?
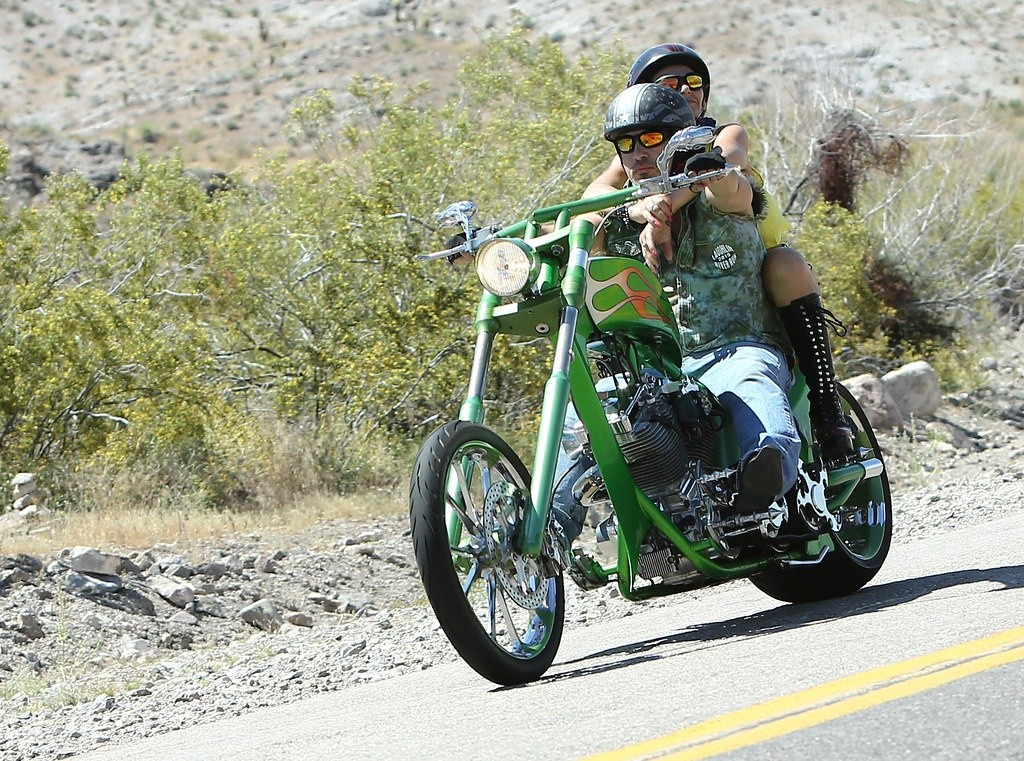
[615,204,630,223]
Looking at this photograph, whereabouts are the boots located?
[779,293,856,465]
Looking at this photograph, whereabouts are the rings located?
[643,243,647,247]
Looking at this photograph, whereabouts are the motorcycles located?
[377,125,892,686]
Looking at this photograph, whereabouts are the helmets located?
[626,43,710,118]
[604,83,696,141]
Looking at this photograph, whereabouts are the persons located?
[549,83,802,548]
[580,43,856,465]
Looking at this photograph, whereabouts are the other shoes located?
[733,445,782,513]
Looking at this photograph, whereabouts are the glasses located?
[653,72,704,91]
[616,129,675,154]
